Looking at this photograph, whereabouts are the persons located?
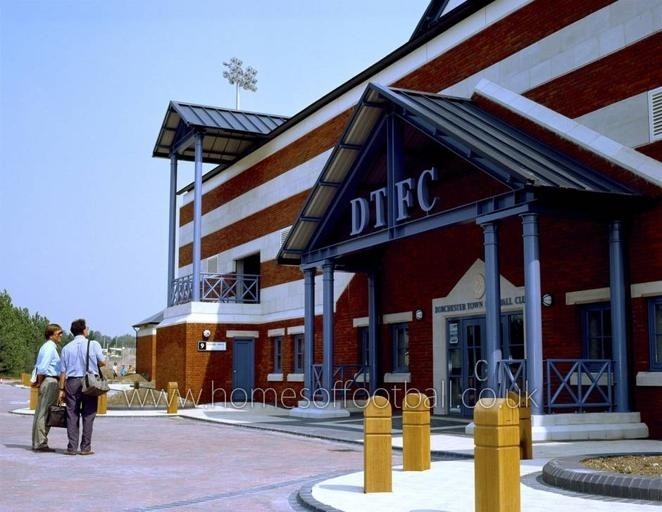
[111,361,136,380]
[28,323,63,454]
[58,318,107,457]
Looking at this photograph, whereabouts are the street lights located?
[222,55,258,110]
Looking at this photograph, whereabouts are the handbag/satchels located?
[81,371,110,396]
[46,406,67,428]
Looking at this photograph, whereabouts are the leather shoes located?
[35,445,56,452]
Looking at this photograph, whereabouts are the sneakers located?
[80,451,95,454]
[63,450,77,455]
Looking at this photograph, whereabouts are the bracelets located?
[57,387,65,392]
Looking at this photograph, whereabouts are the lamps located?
[541,291,553,309]
[413,303,426,321]
